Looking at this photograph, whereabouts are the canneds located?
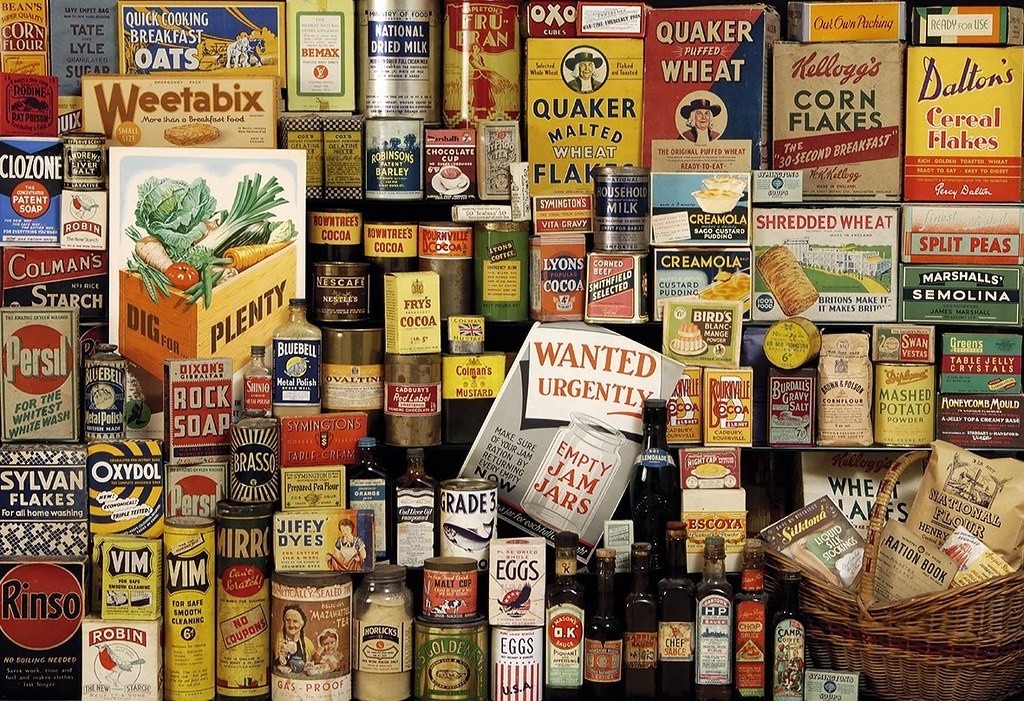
[162,0,652,701]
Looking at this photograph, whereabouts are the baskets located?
[804,629,1024,701]
[762,450,1024,662]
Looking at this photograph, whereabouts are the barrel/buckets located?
[63,2,652,699]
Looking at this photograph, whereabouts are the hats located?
[565,52,604,70]
[680,99,721,119]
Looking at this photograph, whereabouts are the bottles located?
[772,567,807,698]
[736,539,772,701]
[586,547,625,700]
[654,522,695,700]
[695,538,736,701]
[393,446,438,569]
[347,437,391,560]
[84,344,125,442]
[546,530,584,697]
[623,542,655,698]
[352,566,415,698]
[243,346,272,417]
[271,299,325,416]
